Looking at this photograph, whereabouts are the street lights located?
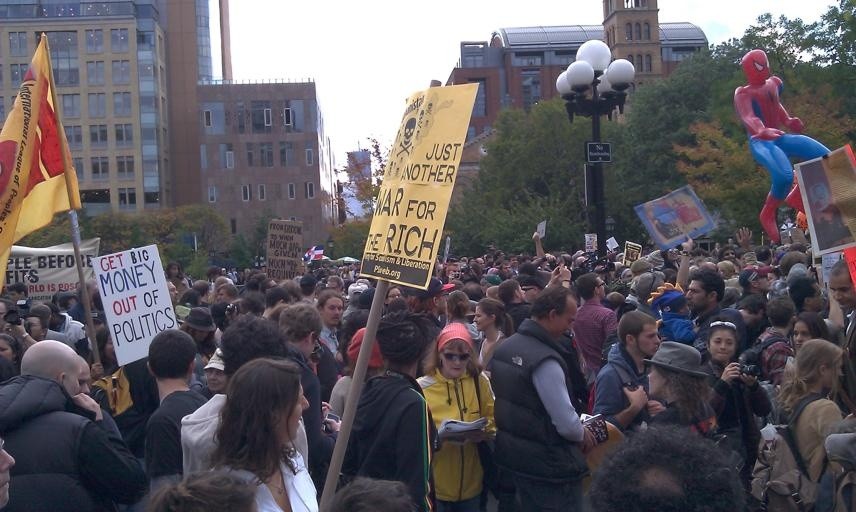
[326,236,335,259]
[554,38,636,257]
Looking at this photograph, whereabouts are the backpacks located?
[738,335,787,381]
[751,393,828,512]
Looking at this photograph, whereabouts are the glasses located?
[442,351,471,363]
[724,252,735,256]
[667,248,679,253]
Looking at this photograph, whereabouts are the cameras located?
[449,271,461,281]
[733,362,761,383]
[224,303,239,314]
[4,310,30,327]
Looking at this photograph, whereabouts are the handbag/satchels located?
[701,432,749,510]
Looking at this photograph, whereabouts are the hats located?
[416,277,455,300]
[349,328,385,368]
[647,282,687,320]
[183,306,217,332]
[345,282,369,301]
[575,256,590,266]
[630,258,655,279]
[204,347,224,371]
[739,265,773,286]
[437,323,473,353]
[642,340,710,379]
[84,311,105,325]
[483,274,502,285]
[521,276,545,289]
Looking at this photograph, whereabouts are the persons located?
[731,48,836,244]
[0,209,856,510]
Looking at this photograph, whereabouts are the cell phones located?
[323,413,340,432]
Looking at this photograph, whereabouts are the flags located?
[0,32,82,295]
